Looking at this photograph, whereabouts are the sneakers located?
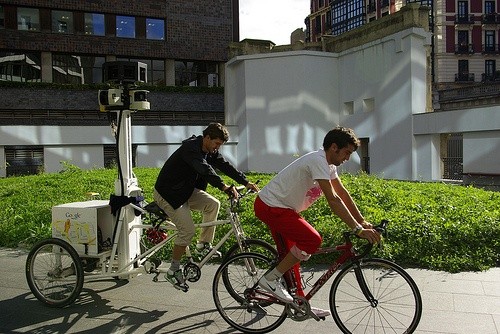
[294,306,330,318]
[164,268,190,292]
[194,242,222,258]
[259,275,294,304]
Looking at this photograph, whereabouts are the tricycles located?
[24,179,284,307]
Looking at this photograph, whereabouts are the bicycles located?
[212,219,422,334]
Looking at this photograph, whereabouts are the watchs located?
[355,225,363,235]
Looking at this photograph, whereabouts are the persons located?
[153,123,261,293]
[254,127,382,319]
